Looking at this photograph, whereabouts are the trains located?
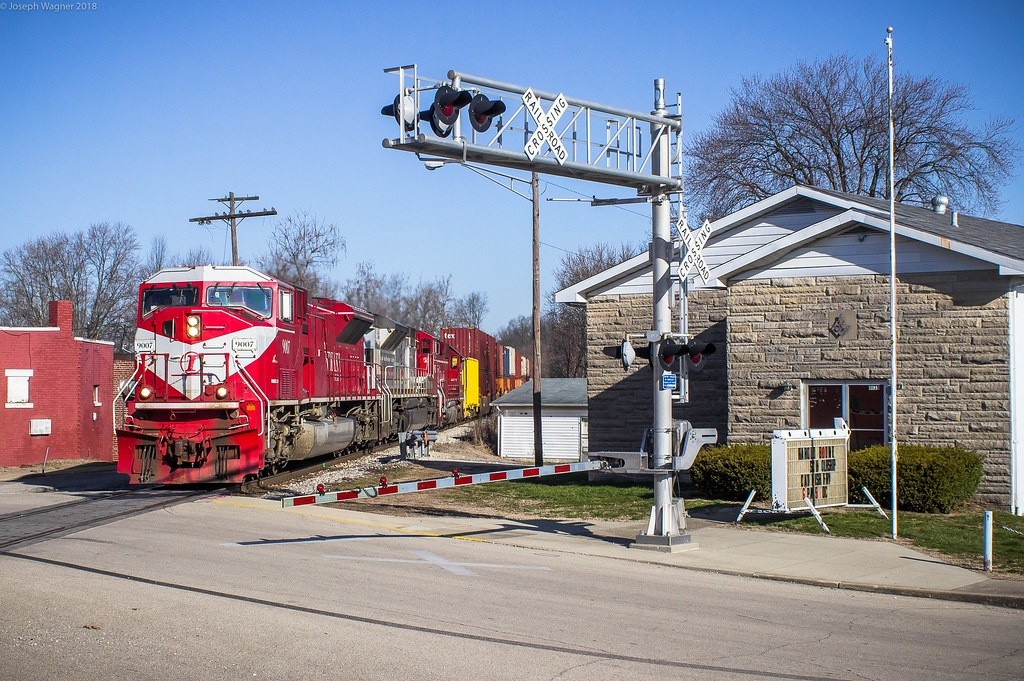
[117,265,531,486]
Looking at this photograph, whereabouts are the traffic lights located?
[468,94,507,132]
[660,339,691,371]
[635,341,652,370]
[434,85,474,126]
[380,93,420,131]
[686,337,717,372]
[602,340,635,371]
[419,102,452,138]
